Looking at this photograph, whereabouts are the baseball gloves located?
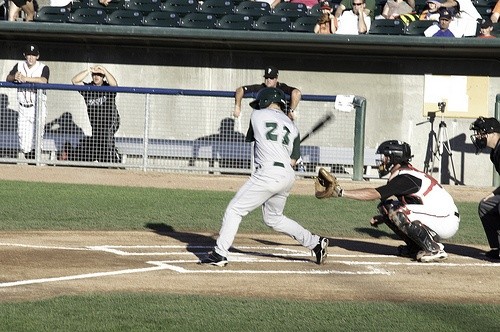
[314,167,343,198]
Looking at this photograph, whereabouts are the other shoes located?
[417,249,448,263]
[38,155,46,166]
[477,250,500,263]
[397,243,446,256]
[120,154,127,163]
[16,153,27,166]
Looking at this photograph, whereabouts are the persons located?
[71,66,124,169]
[334,0,371,35]
[316,140,461,261]
[313,1,338,34]
[470,117,500,259]
[199,88,329,266]
[251,0,329,9]
[419,0,482,38]
[8,0,34,22]
[6,45,49,166]
[478,18,496,38]
[374,0,415,20]
[233,66,301,120]
[99,0,111,6]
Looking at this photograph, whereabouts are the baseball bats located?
[300,111,335,144]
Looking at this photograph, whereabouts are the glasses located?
[265,76,275,80]
[352,3,364,6]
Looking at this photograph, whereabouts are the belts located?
[255,162,285,169]
[19,103,33,108]
[454,212,460,217]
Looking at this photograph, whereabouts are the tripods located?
[431,107,458,185]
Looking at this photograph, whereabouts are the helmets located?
[375,140,411,179]
[249,88,286,110]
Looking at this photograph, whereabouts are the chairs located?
[33,0,500,37]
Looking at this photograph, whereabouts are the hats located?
[479,17,492,28]
[24,44,38,56]
[439,9,451,20]
[484,115,500,133]
[264,66,278,79]
[320,4,331,10]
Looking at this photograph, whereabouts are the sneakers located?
[311,237,329,264]
[196,249,228,267]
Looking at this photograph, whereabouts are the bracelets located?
[290,107,293,112]
